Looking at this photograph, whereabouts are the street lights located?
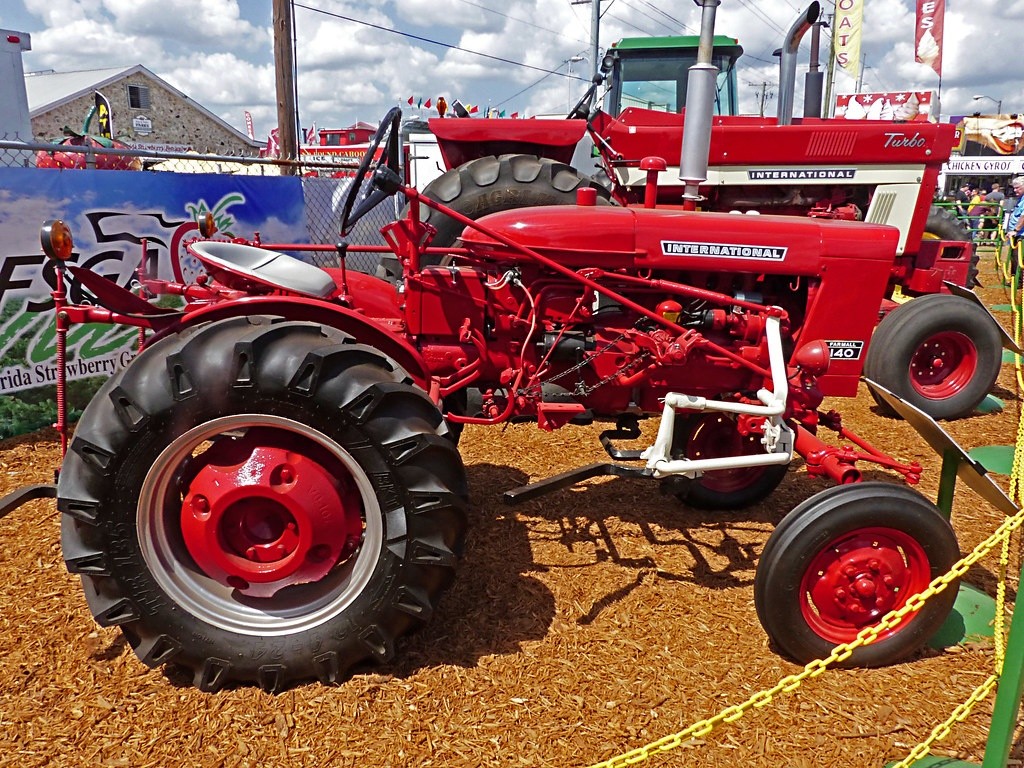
[567,56,584,119]
[972,94,1002,115]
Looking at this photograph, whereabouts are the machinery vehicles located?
[1,0,1004,700]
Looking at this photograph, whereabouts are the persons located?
[1006,176,1024,289]
[932,183,1005,246]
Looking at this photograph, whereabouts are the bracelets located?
[1013,227,1021,232]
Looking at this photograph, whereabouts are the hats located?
[982,190,986,193]
[950,187,955,190]
[965,183,972,187]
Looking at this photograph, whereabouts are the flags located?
[407,95,414,105]
[483,106,487,118]
[510,112,518,117]
[424,98,432,108]
[465,105,471,111]
[417,97,422,109]
[489,111,493,118]
[499,109,506,117]
[305,122,317,145]
[469,105,479,115]
